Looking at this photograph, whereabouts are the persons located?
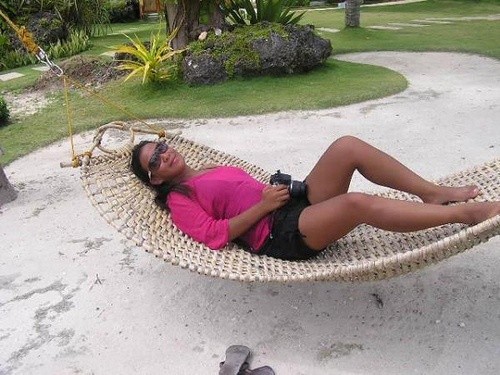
[130,135,500,258]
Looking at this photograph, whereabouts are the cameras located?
[270,170,306,198]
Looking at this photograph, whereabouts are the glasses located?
[147,142,168,181]
